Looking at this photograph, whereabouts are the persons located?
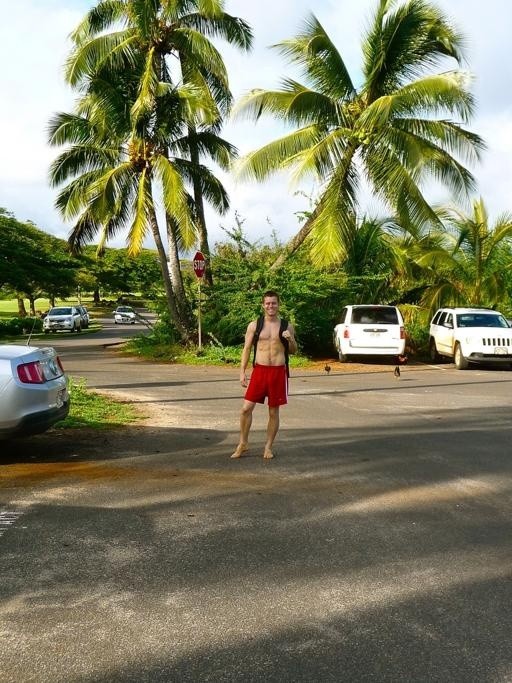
[230,290,298,460]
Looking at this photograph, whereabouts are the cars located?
[428,307,512,370]
[0,346,70,441]
[332,304,409,364]
[43,306,89,333]
[113,306,136,324]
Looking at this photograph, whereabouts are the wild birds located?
[393,366,400,377]
[323,363,331,374]
